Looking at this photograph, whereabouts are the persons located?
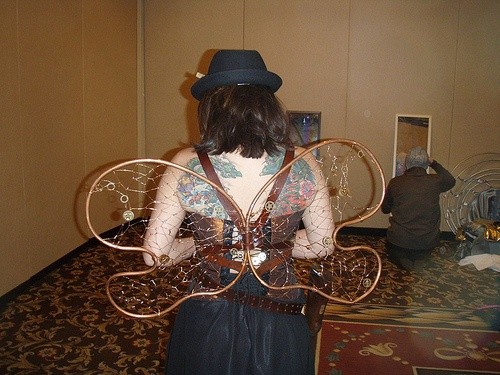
[381,146,457,271]
[143,49,336,375]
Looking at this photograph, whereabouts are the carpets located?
[314,319,500,375]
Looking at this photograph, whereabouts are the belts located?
[198,273,304,315]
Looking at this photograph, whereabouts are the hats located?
[191,49,283,101]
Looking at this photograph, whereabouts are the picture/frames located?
[286,110,321,160]
[393,114,432,179]
